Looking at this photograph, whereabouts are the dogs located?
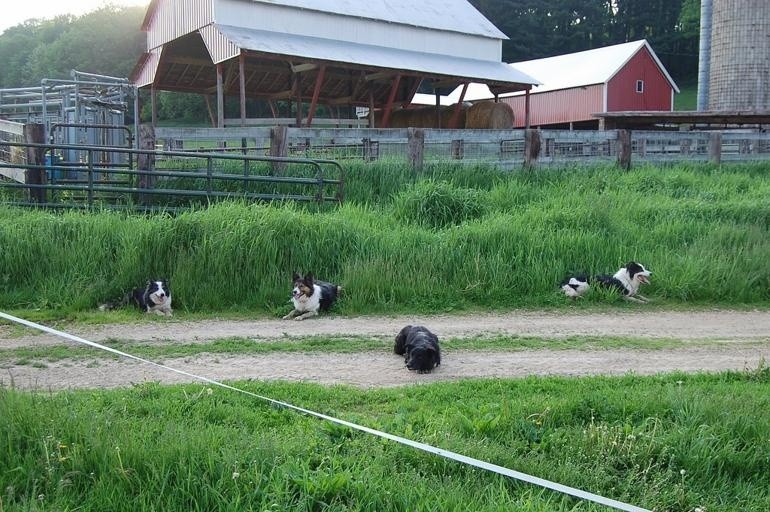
[393,325,441,375]
[98,277,174,318]
[282,270,342,320]
[559,260,653,305]
